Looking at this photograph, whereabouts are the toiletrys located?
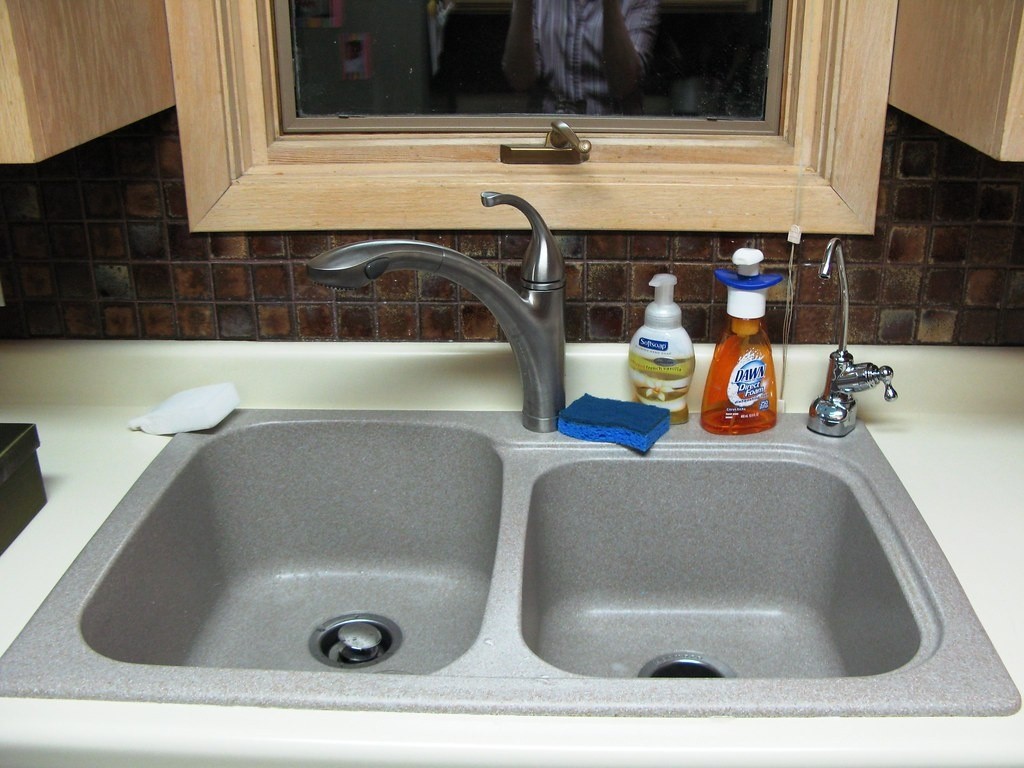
[628,272,696,425]
[700,248,778,433]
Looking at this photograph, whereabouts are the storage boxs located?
[0,423,48,555]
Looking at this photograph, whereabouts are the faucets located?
[805,236,899,437]
[309,195,566,429]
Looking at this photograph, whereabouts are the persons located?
[501,0,661,113]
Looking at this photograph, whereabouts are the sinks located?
[522,409,1023,716]
[0,409,503,714]
